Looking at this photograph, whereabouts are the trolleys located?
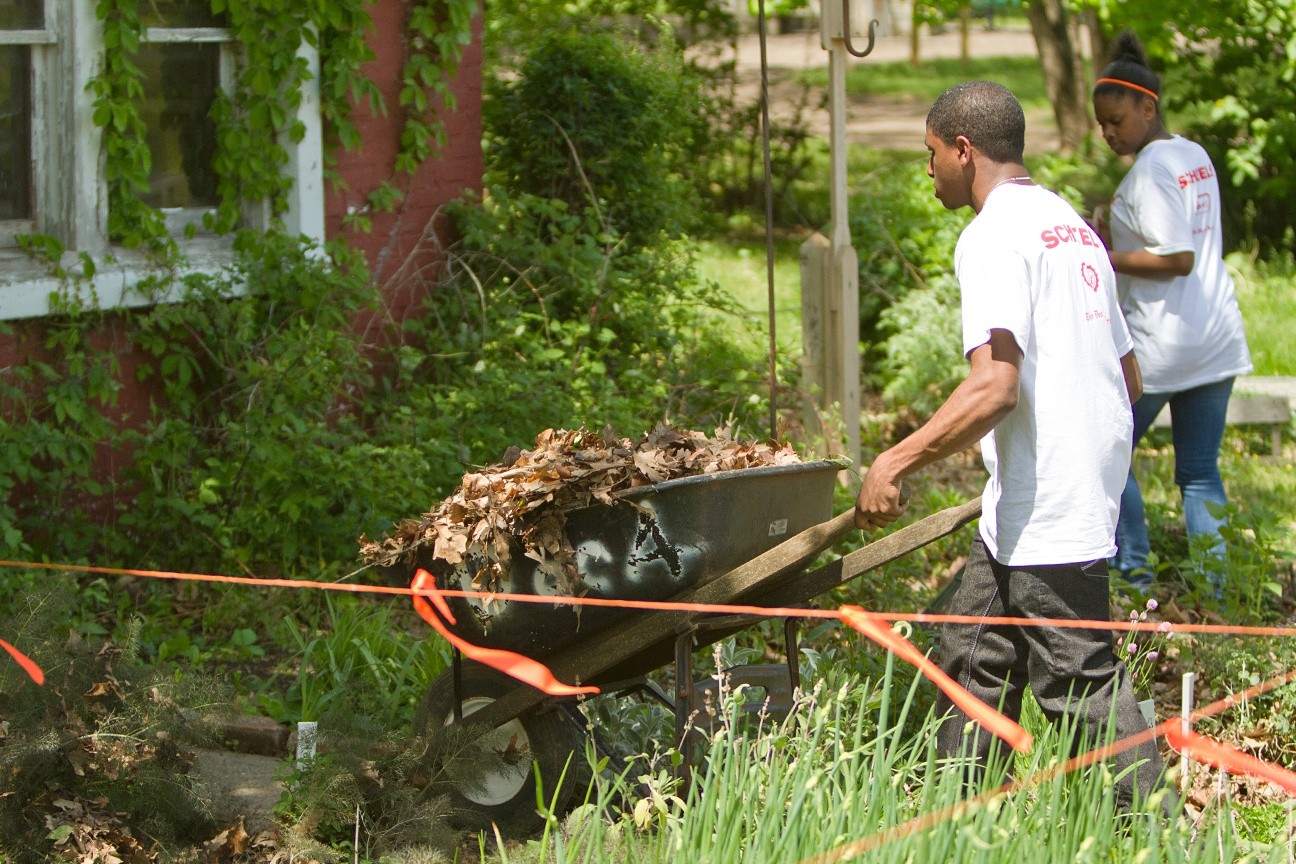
[384,458,983,841]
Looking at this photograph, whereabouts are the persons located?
[852,77,1182,833]
[1066,33,1256,606]
